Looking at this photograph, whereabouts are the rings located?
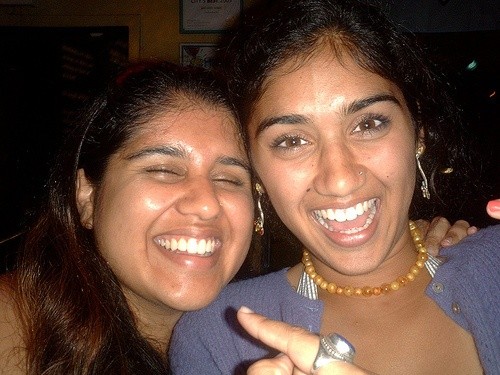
[311,333,354,370]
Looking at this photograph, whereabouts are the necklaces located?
[302,221,430,297]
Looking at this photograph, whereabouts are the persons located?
[0,61,479,374]
[167,1,500,375]
